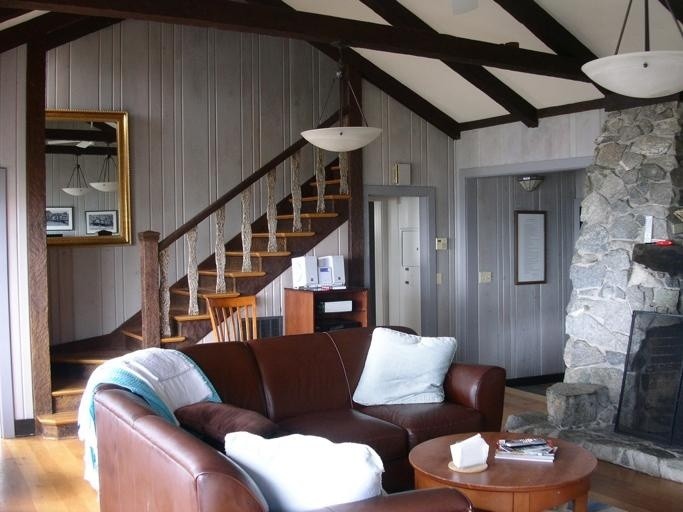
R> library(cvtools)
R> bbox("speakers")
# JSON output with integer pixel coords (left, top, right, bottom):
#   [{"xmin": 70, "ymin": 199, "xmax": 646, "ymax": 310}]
[
  {"xmin": 318, "ymin": 256, "xmax": 345, "ymax": 287},
  {"xmin": 291, "ymin": 256, "xmax": 319, "ymax": 289}
]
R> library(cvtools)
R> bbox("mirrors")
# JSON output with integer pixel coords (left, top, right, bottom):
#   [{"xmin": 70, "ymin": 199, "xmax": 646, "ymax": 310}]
[{"xmin": 42, "ymin": 105, "xmax": 133, "ymax": 250}]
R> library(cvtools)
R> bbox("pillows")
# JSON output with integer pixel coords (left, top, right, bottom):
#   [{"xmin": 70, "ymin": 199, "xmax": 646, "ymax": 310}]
[
  {"xmin": 172, "ymin": 403, "xmax": 278, "ymax": 449},
  {"xmin": 217, "ymin": 425, "xmax": 386, "ymax": 511},
  {"xmin": 348, "ymin": 324, "xmax": 458, "ymax": 412}
]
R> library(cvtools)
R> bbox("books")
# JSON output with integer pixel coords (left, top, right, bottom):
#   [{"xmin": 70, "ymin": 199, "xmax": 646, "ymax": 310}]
[{"xmin": 494, "ymin": 437, "xmax": 557, "ymax": 463}]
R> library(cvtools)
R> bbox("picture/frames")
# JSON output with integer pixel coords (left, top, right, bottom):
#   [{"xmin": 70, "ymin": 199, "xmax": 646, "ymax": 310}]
[
  {"xmin": 511, "ymin": 209, "xmax": 547, "ymax": 287},
  {"xmin": 84, "ymin": 208, "xmax": 119, "ymax": 236},
  {"xmin": 42, "ymin": 207, "xmax": 75, "ymax": 233}
]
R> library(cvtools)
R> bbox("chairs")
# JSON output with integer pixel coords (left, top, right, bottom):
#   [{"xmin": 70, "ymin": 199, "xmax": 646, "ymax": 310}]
[{"xmin": 203, "ymin": 294, "xmax": 257, "ymax": 343}]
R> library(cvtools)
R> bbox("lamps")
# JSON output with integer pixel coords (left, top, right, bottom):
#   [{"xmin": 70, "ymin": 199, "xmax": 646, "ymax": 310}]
[
  {"xmin": 517, "ymin": 174, "xmax": 544, "ymax": 192},
  {"xmin": 300, "ymin": 56, "xmax": 384, "ymax": 155},
  {"xmin": 86, "ymin": 142, "xmax": 118, "ymax": 196},
  {"xmin": 578, "ymin": 0, "xmax": 682, "ymax": 104},
  {"xmin": 57, "ymin": 149, "xmax": 88, "ymax": 198}
]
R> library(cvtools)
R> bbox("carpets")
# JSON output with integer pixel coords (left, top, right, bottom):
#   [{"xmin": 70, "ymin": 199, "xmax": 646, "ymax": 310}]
[{"xmin": 557, "ymin": 483, "xmax": 646, "ymax": 512}]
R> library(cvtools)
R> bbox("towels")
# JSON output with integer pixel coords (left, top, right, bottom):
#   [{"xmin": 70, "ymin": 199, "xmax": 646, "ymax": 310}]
[{"xmin": 69, "ymin": 342, "xmax": 221, "ymax": 493}]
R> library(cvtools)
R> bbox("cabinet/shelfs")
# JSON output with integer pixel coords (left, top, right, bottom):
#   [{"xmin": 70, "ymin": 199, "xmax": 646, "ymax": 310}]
[{"xmin": 282, "ymin": 284, "xmax": 371, "ymax": 336}]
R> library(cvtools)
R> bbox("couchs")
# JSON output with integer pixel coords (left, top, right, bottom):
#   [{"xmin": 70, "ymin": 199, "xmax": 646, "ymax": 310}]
[{"xmin": 92, "ymin": 325, "xmax": 509, "ymax": 512}]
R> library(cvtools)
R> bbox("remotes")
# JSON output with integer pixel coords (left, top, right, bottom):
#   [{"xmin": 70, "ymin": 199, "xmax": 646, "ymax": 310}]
[{"xmin": 505, "ymin": 437, "xmax": 548, "ymax": 448}]
